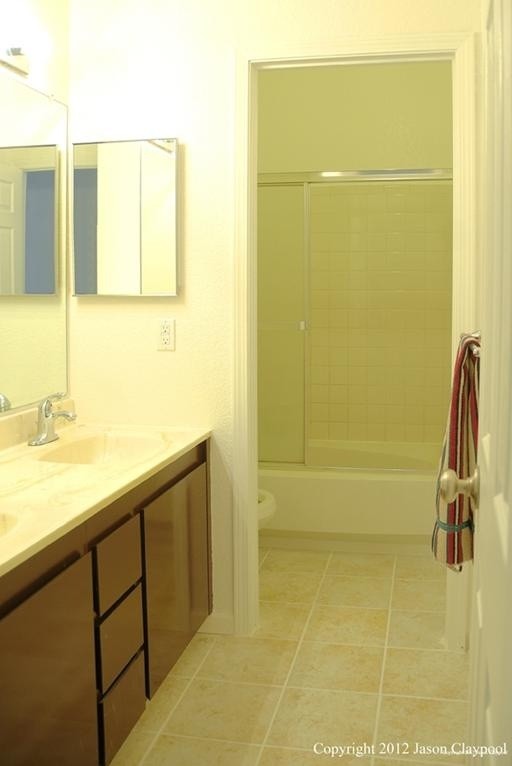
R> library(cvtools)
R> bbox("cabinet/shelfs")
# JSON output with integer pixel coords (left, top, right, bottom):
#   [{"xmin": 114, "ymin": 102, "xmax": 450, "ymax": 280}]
[{"xmin": 0, "ymin": 461, "xmax": 214, "ymax": 766}]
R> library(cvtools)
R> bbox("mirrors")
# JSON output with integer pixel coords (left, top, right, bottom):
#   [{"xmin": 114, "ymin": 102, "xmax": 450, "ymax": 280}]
[
  {"xmin": 0, "ymin": 145, "xmax": 61, "ymax": 296},
  {"xmin": 0, "ymin": 73, "xmax": 69, "ymax": 417},
  {"xmin": 74, "ymin": 137, "xmax": 178, "ymax": 298}
]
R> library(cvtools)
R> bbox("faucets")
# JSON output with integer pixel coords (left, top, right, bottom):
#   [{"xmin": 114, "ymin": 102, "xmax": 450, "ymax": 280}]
[
  {"xmin": 28, "ymin": 394, "xmax": 76, "ymax": 445},
  {"xmin": 0, "ymin": 394, "xmax": 9, "ymax": 412}
]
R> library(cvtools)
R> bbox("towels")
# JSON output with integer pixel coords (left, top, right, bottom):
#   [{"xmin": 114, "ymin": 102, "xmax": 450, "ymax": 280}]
[{"xmin": 431, "ymin": 334, "xmax": 479, "ymax": 573}]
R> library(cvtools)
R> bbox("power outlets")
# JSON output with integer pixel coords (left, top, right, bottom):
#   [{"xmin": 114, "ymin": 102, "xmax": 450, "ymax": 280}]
[{"xmin": 156, "ymin": 319, "xmax": 175, "ymax": 351}]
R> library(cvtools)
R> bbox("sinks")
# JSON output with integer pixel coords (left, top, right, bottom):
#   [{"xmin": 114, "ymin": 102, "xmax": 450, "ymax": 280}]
[{"xmin": 37, "ymin": 432, "xmax": 164, "ymax": 465}]
